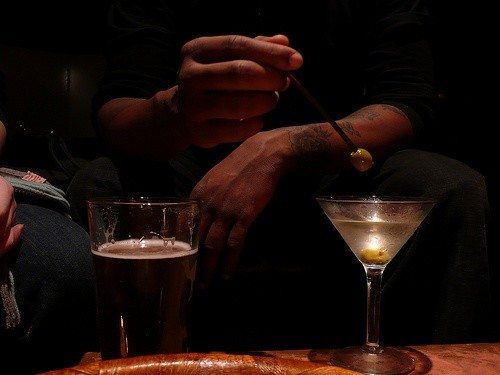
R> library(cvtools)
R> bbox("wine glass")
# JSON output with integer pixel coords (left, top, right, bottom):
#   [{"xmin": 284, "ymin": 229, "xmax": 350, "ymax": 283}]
[{"xmin": 316, "ymin": 195, "xmax": 438, "ymax": 375}]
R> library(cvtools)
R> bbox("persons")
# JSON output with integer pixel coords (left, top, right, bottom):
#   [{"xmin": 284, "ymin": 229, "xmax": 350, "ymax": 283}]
[
  {"xmin": 65, "ymin": 1, "xmax": 493, "ymax": 355},
  {"xmin": 1, "ymin": 118, "xmax": 99, "ymax": 375}
]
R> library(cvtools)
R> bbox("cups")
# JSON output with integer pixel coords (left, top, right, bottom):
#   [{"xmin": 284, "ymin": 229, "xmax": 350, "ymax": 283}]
[{"xmin": 86, "ymin": 195, "xmax": 205, "ymax": 359}]
